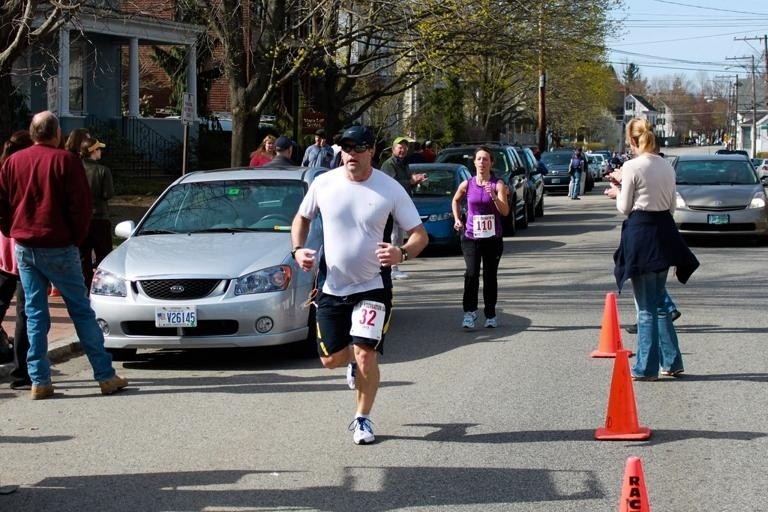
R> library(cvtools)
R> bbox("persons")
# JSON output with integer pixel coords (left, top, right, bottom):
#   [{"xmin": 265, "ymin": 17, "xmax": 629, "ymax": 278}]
[
  {"xmin": 605, "ymin": 167, "xmax": 681, "ymax": 334},
  {"xmin": 452, "ymin": 147, "xmax": 510, "ymax": 328},
  {"xmin": 604, "ymin": 117, "xmax": 700, "ymax": 381},
  {"xmin": 568, "ymin": 147, "xmax": 587, "ymax": 200},
  {"xmin": 0, "ymin": 111, "xmax": 128, "ymax": 400},
  {"xmin": 380, "ymin": 137, "xmax": 428, "ymax": 280},
  {"xmin": 250, "ymin": 129, "xmax": 435, "ymax": 171},
  {"xmin": 290, "ymin": 127, "xmax": 429, "ymax": 445}
]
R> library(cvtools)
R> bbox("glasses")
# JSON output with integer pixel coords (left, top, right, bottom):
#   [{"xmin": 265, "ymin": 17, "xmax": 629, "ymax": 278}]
[{"xmin": 340, "ymin": 143, "xmax": 369, "ymax": 153}]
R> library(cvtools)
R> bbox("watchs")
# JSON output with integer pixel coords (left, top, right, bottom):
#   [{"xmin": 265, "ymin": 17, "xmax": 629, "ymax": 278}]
[{"xmin": 291, "ymin": 247, "xmax": 301, "ymax": 259}]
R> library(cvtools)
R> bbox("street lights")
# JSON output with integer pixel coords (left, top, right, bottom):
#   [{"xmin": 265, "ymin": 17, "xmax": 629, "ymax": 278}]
[
  {"xmin": 703, "ymin": 95, "xmax": 730, "ymax": 143},
  {"xmin": 724, "ymin": 63, "xmax": 756, "ymax": 158},
  {"xmin": 714, "ymin": 75, "xmax": 739, "ymax": 151}
]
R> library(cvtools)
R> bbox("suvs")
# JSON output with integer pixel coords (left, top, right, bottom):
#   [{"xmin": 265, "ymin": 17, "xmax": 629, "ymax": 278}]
[
  {"xmin": 505, "ymin": 143, "xmax": 549, "ymax": 223},
  {"xmin": 540, "ymin": 146, "xmax": 595, "ymax": 193},
  {"xmin": 434, "ymin": 140, "xmax": 530, "ymax": 235}
]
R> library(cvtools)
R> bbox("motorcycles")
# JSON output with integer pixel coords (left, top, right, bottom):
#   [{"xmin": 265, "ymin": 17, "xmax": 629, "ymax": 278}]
[{"xmin": 688, "ymin": 138, "xmax": 694, "ymax": 145}]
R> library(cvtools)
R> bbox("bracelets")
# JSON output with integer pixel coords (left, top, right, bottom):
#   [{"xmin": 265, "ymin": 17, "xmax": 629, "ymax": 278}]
[
  {"xmin": 492, "ymin": 196, "xmax": 498, "ymax": 201},
  {"xmin": 400, "ymin": 248, "xmax": 408, "ymax": 262}
]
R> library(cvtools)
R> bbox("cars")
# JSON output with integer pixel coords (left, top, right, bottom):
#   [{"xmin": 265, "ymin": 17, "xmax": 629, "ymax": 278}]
[
  {"xmin": 670, "ymin": 149, "xmax": 768, "ymax": 243},
  {"xmin": 587, "ymin": 149, "xmax": 612, "ymax": 182},
  {"xmin": 88, "ymin": 165, "xmax": 330, "ymax": 360},
  {"xmin": 407, "ymin": 162, "xmax": 474, "ymax": 252}
]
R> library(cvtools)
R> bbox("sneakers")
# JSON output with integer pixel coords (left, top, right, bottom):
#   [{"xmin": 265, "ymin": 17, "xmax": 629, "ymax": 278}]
[
  {"xmin": 10, "ymin": 380, "xmax": 55, "ymax": 399},
  {"xmin": 672, "ymin": 310, "xmax": 680, "ymax": 320},
  {"xmin": 100, "ymin": 374, "xmax": 129, "ymax": 393},
  {"xmin": 391, "ymin": 266, "xmax": 409, "ymax": 280},
  {"xmin": 631, "ymin": 373, "xmax": 658, "ymax": 380},
  {"xmin": 484, "ymin": 318, "xmax": 497, "ymax": 328},
  {"xmin": 626, "ymin": 325, "xmax": 637, "ymax": 333},
  {"xmin": 462, "ymin": 309, "xmax": 479, "ymax": 328},
  {"xmin": 661, "ymin": 369, "xmax": 684, "ymax": 375},
  {"xmin": 347, "ymin": 361, "xmax": 357, "ymax": 389},
  {"xmin": 48, "ymin": 286, "xmax": 61, "ymax": 296},
  {"xmin": 348, "ymin": 417, "xmax": 375, "ymax": 445}
]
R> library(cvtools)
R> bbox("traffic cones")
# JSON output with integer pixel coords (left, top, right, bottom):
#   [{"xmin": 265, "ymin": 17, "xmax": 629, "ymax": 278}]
[
  {"xmin": 618, "ymin": 457, "xmax": 649, "ymax": 512},
  {"xmin": 588, "ymin": 292, "xmax": 634, "ymax": 360},
  {"xmin": 594, "ymin": 349, "xmax": 652, "ymax": 443}
]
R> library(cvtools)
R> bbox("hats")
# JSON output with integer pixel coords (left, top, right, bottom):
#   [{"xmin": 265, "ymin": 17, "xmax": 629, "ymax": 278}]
[
  {"xmin": 315, "ymin": 129, "xmax": 327, "ymax": 136},
  {"xmin": 275, "ymin": 136, "xmax": 296, "ymax": 148},
  {"xmin": 88, "ymin": 139, "xmax": 106, "ymax": 153},
  {"xmin": 393, "ymin": 137, "xmax": 409, "ymax": 147},
  {"xmin": 338, "ymin": 126, "xmax": 374, "ymax": 147}
]
